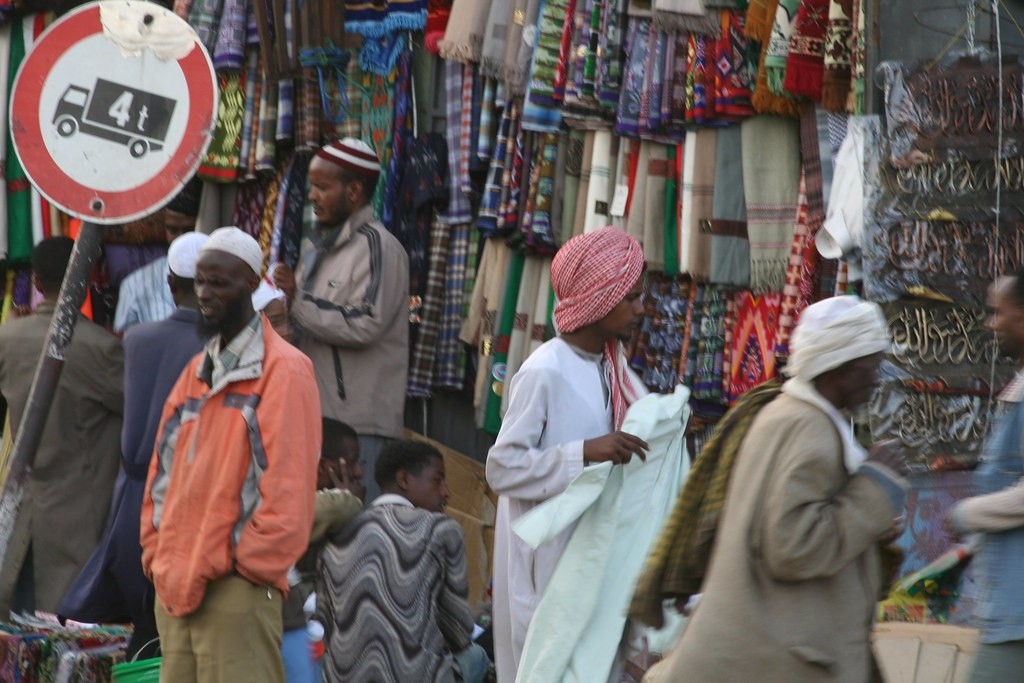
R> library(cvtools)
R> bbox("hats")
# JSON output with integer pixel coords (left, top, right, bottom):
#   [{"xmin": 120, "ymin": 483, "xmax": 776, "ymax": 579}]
[
  {"xmin": 201, "ymin": 224, "xmax": 264, "ymax": 277},
  {"xmin": 787, "ymin": 294, "xmax": 889, "ymax": 379},
  {"xmin": 167, "ymin": 231, "xmax": 210, "ymax": 279},
  {"xmin": 315, "ymin": 136, "xmax": 381, "ymax": 178},
  {"xmin": 548, "ymin": 227, "xmax": 646, "ymax": 336}
]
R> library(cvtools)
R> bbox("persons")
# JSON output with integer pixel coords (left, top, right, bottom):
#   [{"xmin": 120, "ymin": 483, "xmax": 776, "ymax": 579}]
[
  {"xmin": 946, "ymin": 272, "xmax": 1024, "ymax": 683},
  {"xmin": 486, "ymin": 228, "xmax": 694, "ymax": 683},
  {"xmin": 640, "ymin": 296, "xmax": 910, "ymax": 683},
  {"xmin": 0, "ymin": 135, "xmax": 487, "ymax": 683}
]
